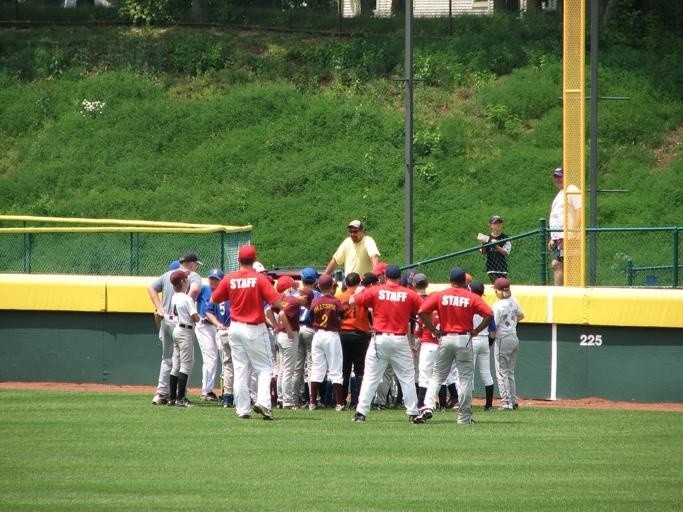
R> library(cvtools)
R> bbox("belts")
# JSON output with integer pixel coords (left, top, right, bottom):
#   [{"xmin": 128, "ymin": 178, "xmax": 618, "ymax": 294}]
[
  {"xmin": 376, "ymin": 332, "xmax": 406, "ymax": 335},
  {"xmin": 442, "ymin": 333, "xmax": 467, "ymax": 335},
  {"xmin": 179, "ymin": 325, "xmax": 192, "ymax": 329}
]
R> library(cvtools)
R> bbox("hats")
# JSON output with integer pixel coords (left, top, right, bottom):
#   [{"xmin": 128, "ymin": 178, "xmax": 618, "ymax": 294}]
[
  {"xmin": 277, "ymin": 268, "xmax": 337, "ymax": 310},
  {"xmin": 169, "ymin": 252, "xmax": 202, "ymax": 285},
  {"xmin": 491, "ymin": 278, "xmax": 510, "ymax": 291},
  {"xmin": 554, "ymin": 168, "xmax": 563, "ymax": 176},
  {"xmin": 348, "ymin": 220, "xmax": 363, "ymax": 230},
  {"xmin": 239, "ymin": 245, "xmax": 256, "ymax": 259},
  {"xmin": 346, "ymin": 263, "xmax": 428, "ymax": 287},
  {"xmin": 253, "ymin": 262, "xmax": 268, "ymax": 272},
  {"xmin": 490, "ymin": 216, "xmax": 502, "ymax": 223},
  {"xmin": 209, "ymin": 269, "xmax": 223, "ymax": 280},
  {"xmin": 450, "ymin": 267, "xmax": 483, "ymax": 294}
]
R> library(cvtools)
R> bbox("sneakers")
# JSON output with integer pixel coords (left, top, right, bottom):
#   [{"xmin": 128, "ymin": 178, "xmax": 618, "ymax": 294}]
[
  {"xmin": 409, "ymin": 408, "xmax": 433, "ymax": 423},
  {"xmin": 498, "ymin": 404, "xmax": 518, "ymax": 410},
  {"xmin": 200, "ymin": 393, "xmax": 233, "ymax": 407},
  {"xmin": 152, "ymin": 393, "xmax": 191, "ymax": 406},
  {"xmin": 458, "ymin": 419, "xmax": 475, "ymax": 424},
  {"xmin": 277, "ymin": 398, "xmax": 347, "ymax": 411},
  {"xmin": 352, "ymin": 415, "xmax": 365, "ymax": 421},
  {"xmin": 253, "ymin": 404, "xmax": 271, "ymax": 417}
]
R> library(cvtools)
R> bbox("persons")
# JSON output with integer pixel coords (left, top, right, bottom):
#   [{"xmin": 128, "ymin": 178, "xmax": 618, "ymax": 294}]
[
  {"xmin": 479, "ymin": 216, "xmax": 512, "ymax": 281},
  {"xmin": 149, "ymin": 245, "xmax": 523, "ymax": 423},
  {"xmin": 548, "ymin": 168, "xmax": 584, "ymax": 286},
  {"xmin": 325, "ymin": 220, "xmax": 380, "ymax": 274}
]
